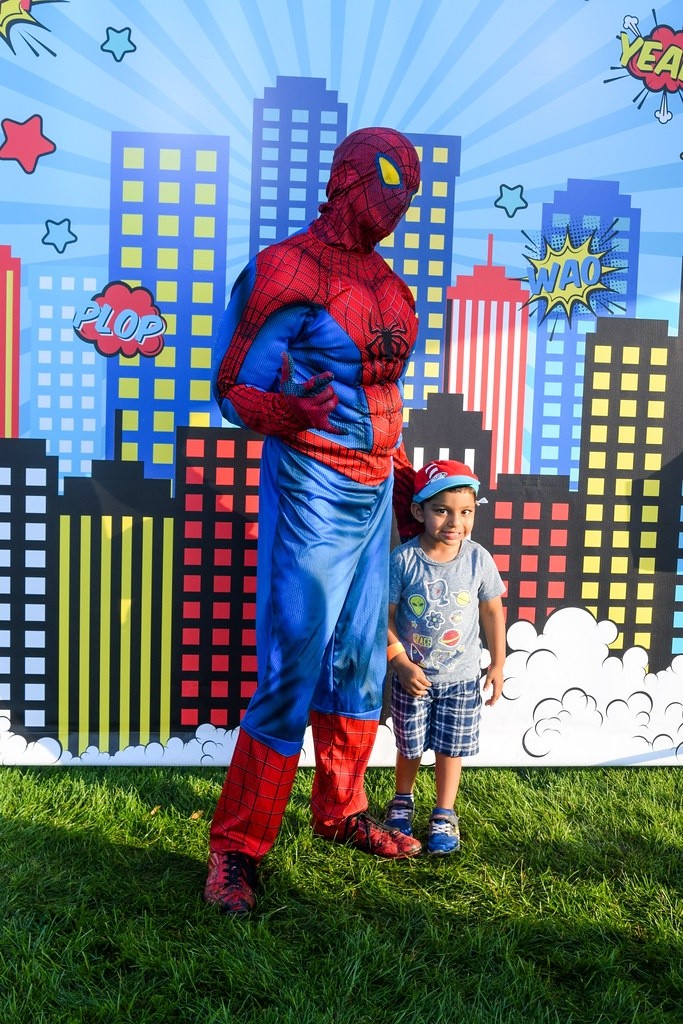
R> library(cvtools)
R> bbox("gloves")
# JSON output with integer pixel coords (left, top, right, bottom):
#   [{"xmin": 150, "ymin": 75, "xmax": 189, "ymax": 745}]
[{"xmin": 279, "ymin": 352, "xmax": 351, "ymax": 436}]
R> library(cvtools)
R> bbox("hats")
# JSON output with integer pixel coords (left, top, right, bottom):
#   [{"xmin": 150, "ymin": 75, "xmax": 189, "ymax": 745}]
[{"xmin": 412, "ymin": 460, "xmax": 479, "ymax": 504}]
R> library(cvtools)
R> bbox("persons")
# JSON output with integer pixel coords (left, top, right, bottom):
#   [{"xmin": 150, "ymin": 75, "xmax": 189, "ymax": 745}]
[
  {"xmin": 206, "ymin": 124, "xmax": 425, "ymax": 911},
  {"xmin": 385, "ymin": 460, "xmax": 508, "ymax": 855}
]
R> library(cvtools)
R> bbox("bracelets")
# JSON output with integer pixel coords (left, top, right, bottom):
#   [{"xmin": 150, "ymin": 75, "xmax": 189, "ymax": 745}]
[{"xmin": 387, "ymin": 642, "xmax": 405, "ymax": 661}]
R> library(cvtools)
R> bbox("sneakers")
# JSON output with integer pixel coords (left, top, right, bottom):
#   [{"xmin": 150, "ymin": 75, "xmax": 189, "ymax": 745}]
[
  {"xmin": 427, "ymin": 807, "xmax": 459, "ymax": 854},
  {"xmin": 311, "ymin": 811, "xmax": 423, "ymax": 859},
  {"xmin": 385, "ymin": 795, "xmax": 416, "ymax": 838},
  {"xmin": 201, "ymin": 850, "xmax": 261, "ymax": 914}
]
